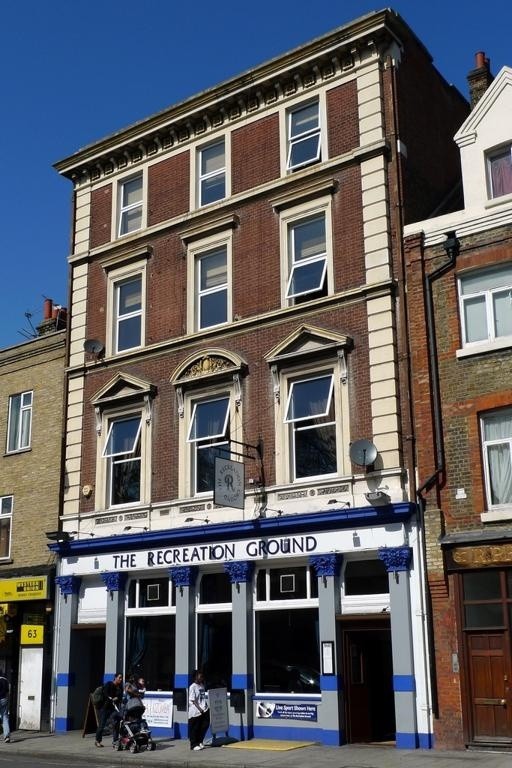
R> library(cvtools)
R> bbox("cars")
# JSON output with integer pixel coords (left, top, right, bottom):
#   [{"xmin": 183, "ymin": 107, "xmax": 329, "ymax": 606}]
[{"xmin": 260, "ymin": 660, "xmax": 322, "ymax": 693}]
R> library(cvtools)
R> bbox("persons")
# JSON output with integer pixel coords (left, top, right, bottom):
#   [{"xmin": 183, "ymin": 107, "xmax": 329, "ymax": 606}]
[
  {"xmin": 0, "ymin": 666, "xmax": 12, "ymax": 745},
  {"xmin": 121, "ymin": 669, "xmax": 145, "ymax": 708},
  {"xmin": 135, "ymin": 677, "xmax": 147, "ymax": 700},
  {"xmin": 188, "ymin": 669, "xmax": 211, "ymax": 753},
  {"xmin": 95, "ymin": 671, "xmax": 123, "ymax": 748}
]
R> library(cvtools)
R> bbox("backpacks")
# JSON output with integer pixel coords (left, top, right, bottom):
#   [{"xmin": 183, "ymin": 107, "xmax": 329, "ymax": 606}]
[{"xmin": 90, "ymin": 686, "xmax": 103, "ymax": 706}]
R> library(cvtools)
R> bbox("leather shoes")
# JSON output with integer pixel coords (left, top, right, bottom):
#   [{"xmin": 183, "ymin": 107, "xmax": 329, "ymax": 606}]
[{"xmin": 94, "ymin": 741, "xmax": 104, "ymax": 747}]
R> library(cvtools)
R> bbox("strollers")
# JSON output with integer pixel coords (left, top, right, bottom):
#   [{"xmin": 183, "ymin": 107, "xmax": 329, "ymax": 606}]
[{"xmin": 109, "ymin": 690, "xmax": 156, "ymax": 755}]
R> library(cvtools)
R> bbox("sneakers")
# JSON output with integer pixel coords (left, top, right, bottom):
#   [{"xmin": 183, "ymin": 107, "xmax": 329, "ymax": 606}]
[
  {"xmin": 192, "ymin": 745, "xmax": 202, "ymax": 751},
  {"xmin": 5, "ymin": 736, "xmax": 11, "ymax": 742},
  {"xmin": 199, "ymin": 743, "xmax": 205, "ymax": 749}
]
